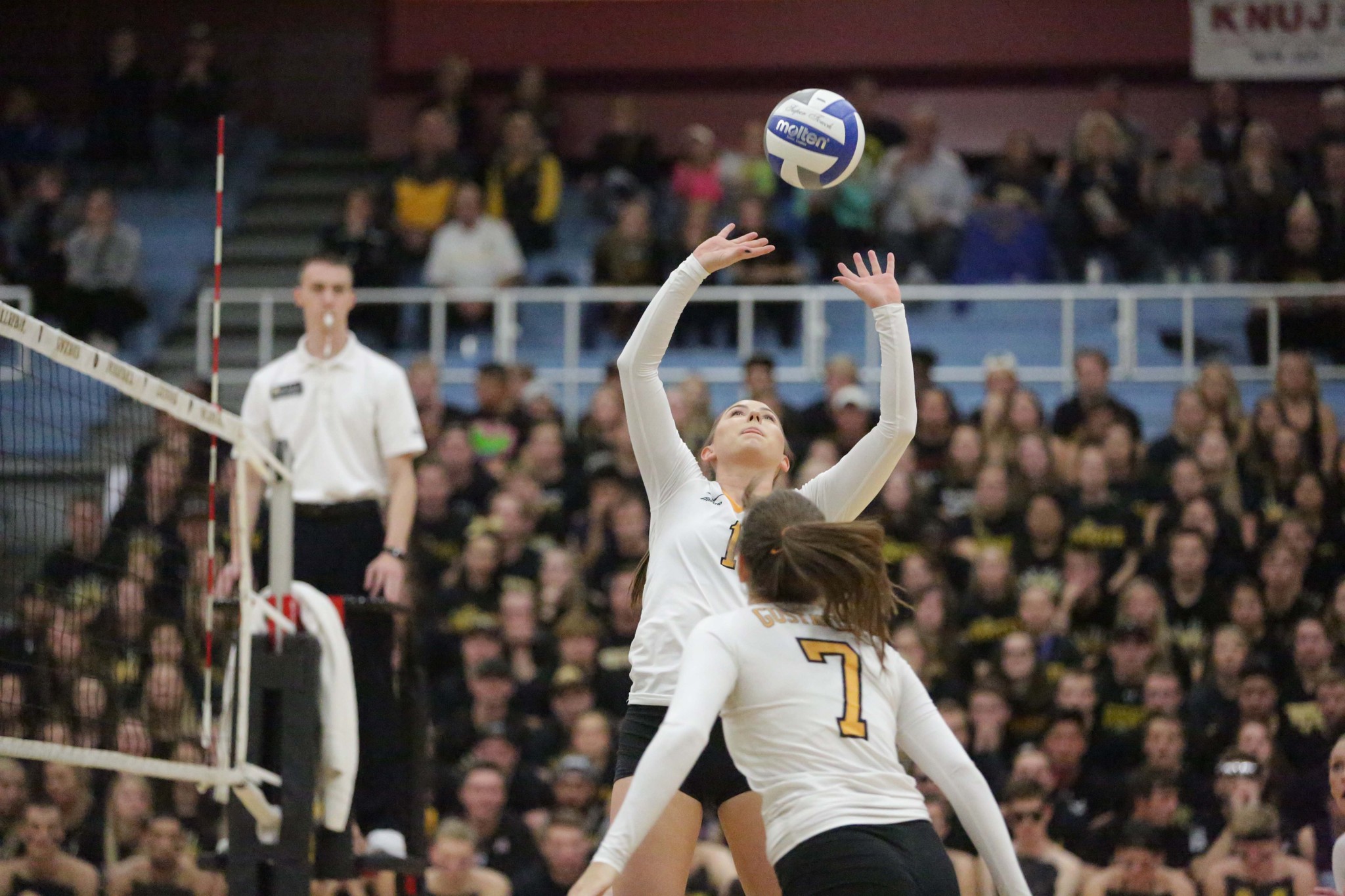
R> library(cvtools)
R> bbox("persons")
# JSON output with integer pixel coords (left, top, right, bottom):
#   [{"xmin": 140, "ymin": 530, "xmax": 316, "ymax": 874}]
[
  {"xmin": 566, "ymin": 485, "xmax": 1036, "ymax": 896},
  {"xmin": 607, "ymin": 222, "xmax": 920, "ymax": 896},
  {"xmin": 215, "ymin": 253, "xmax": 427, "ymax": 863},
  {"xmin": 0, "ymin": 1, "xmax": 1345, "ymax": 896}
]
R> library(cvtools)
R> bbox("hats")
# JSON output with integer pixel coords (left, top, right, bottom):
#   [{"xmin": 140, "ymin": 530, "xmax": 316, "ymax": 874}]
[
  {"xmin": 557, "ymin": 754, "xmax": 591, "ymax": 772},
  {"xmin": 831, "ymin": 385, "xmax": 873, "ymax": 411},
  {"xmin": 365, "ymin": 829, "xmax": 407, "ymax": 860},
  {"xmin": 983, "ymin": 352, "xmax": 1016, "ymax": 375},
  {"xmin": 685, "ymin": 123, "xmax": 713, "ymax": 144},
  {"xmin": 547, "ymin": 660, "xmax": 591, "ymax": 693}
]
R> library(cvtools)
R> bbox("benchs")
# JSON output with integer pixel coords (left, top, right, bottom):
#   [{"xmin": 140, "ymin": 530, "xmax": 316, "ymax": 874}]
[
  {"xmin": 389, "ymin": 184, "xmax": 1345, "ymax": 439},
  {"xmin": 0, "ymin": 117, "xmax": 280, "ymax": 459}
]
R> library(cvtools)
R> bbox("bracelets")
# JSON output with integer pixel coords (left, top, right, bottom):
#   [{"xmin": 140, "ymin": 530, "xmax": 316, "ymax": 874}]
[{"xmin": 381, "ymin": 543, "xmax": 410, "ymax": 562}]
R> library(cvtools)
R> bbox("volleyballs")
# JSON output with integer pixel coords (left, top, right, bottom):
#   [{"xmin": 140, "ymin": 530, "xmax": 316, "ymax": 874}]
[{"xmin": 762, "ymin": 86, "xmax": 867, "ymax": 190}]
